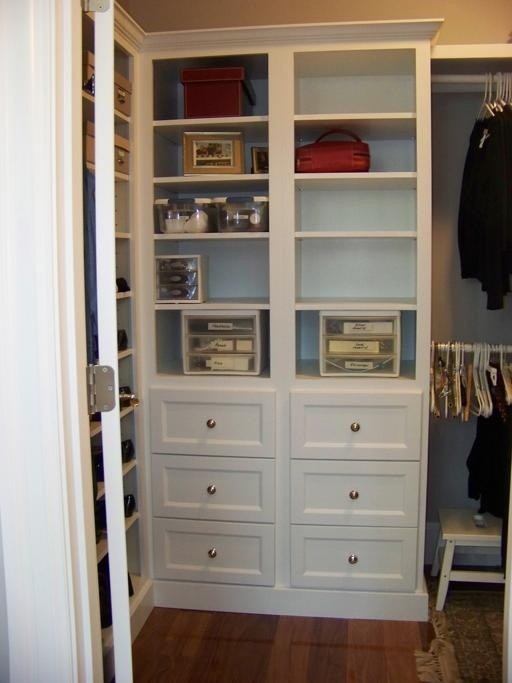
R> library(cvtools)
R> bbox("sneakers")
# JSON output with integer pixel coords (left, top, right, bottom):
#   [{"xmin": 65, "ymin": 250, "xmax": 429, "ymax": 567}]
[{"xmin": 88, "ymin": 275, "xmax": 136, "ymax": 627}]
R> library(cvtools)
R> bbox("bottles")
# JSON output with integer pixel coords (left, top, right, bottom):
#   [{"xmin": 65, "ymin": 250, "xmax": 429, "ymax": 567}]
[
  {"xmin": 166, "ymin": 198, "xmax": 193, "ymax": 221},
  {"xmin": 222, "ymin": 197, "xmax": 253, "ymax": 227}
]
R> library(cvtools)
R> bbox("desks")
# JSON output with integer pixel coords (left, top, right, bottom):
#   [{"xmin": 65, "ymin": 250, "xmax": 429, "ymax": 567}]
[{"xmin": 143, "ymin": 386, "xmax": 288, "ymax": 616}]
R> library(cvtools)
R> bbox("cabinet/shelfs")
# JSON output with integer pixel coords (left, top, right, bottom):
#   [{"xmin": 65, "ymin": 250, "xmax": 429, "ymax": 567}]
[
  {"xmin": 143, "ymin": 40, "xmax": 286, "ymax": 386},
  {"xmin": 2, "ymin": 0, "xmax": 146, "ymax": 683},
  {"xmin": 288, "ymin": 385, "xmax": 428, "ymax": 622},
  {"xmin": 285, "ymin": 39, "xmax": 432, "ymax": 385}
]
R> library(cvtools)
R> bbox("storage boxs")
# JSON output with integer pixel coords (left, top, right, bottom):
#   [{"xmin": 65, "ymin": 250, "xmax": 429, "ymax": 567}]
[
  {"xmin": 180, "ymin": 66, "xmax": 256, "ymax": 118},
  {"xmin": 181, "ymin": 310, "xmax": 261, "ymax": 376},
  {"xmin": 318, "ymin": 310, "xmax": 401, "ymax": 378},
  {"xmin": 155, "ymin": 254, "xmax": 207, "ymax": 304},
  {"xmin": 82, "ymin": 50, "xmax": 95, "ymax": 90},
  {"xmin": 155, "ymin": 196, "xmax": 214, "ymax": 234},
  {"xmin": 113, "ymin": 71, "xmax": 133, "ymax": 116},
  {"xmin": 83, "ymin": 120, "xmax": 95, "ymax": 165},
  {"xmin": 211, "ymin": 197, "xmax": 269, "ymax": 232},
  {"xmin": 114, "ymin": 133, "xmax": 129, "ymax": 174}
]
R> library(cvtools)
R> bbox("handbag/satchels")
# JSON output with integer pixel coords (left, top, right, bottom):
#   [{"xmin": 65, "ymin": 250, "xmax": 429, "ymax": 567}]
[{"xmin": 295, "ymin": 127, "xmax": 370, "ymax": 172}]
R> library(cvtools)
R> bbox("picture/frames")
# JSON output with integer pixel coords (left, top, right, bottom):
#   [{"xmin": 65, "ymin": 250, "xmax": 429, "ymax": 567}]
[
  {"xmin": 182, "ymin": 131, "xmax": 245, "ymax": 176},
  {"xmin": 251, "ymin": 146, "xmax": 269, "ymax": 173}
]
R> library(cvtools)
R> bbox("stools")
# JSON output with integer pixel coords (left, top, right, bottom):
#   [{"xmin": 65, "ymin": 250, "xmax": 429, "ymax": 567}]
[{"xmin": 431, "ymin": 510, "xmax": 506, "ymax": 611}]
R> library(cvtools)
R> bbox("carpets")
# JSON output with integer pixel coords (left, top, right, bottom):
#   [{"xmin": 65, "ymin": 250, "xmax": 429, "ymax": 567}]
[{"xmin": 413, "ymin": 592, "xmax": 505, "ymax": 683}]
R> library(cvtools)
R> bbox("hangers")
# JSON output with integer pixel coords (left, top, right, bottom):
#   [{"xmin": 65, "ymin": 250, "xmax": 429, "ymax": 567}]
[
  {"xmin": 474, "ymin": 71, "xmax": 511, "ymax": 120},
  {"xmin": 429, "ymin": 340, "xmax": 512, "ymax": 422}
]
